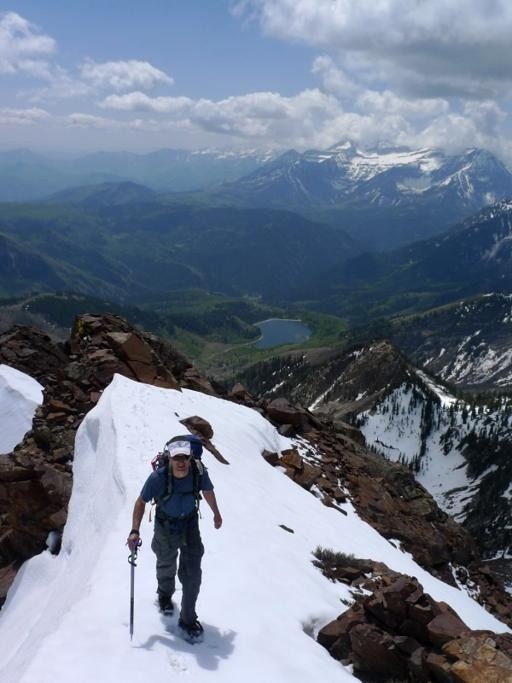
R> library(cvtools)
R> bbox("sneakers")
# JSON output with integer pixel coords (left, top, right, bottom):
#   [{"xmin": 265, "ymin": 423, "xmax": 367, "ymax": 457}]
[
  {"xmin": 158, "ymin": 593, "xmax": 174, "ymax": 616},
  {"xmin": 178, "ymin": 613, "xmax": 204, "ymax": 637}
]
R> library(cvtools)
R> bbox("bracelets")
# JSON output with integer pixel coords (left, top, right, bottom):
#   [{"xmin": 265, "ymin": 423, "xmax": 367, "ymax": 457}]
[{"xmin": 130, "ymin": 529, "xmax": 139, "ymax": 536}]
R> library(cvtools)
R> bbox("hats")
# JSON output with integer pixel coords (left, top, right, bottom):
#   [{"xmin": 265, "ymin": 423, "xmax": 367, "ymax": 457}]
[{"xmin": 167, "ymin": 440, "xmax": 191, "ymax": 458}]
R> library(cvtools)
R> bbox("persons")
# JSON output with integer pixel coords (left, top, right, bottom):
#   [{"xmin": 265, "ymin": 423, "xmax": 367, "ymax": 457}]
[{"xmin": 127, "ymin": 436, "xmax": 222, "ymax": 637}]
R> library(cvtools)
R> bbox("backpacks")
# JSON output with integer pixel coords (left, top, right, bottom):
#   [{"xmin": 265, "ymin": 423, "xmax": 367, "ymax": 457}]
[{"xmin": 150, "ymin": 435, "xmax": 204, "ymax": 523}]
